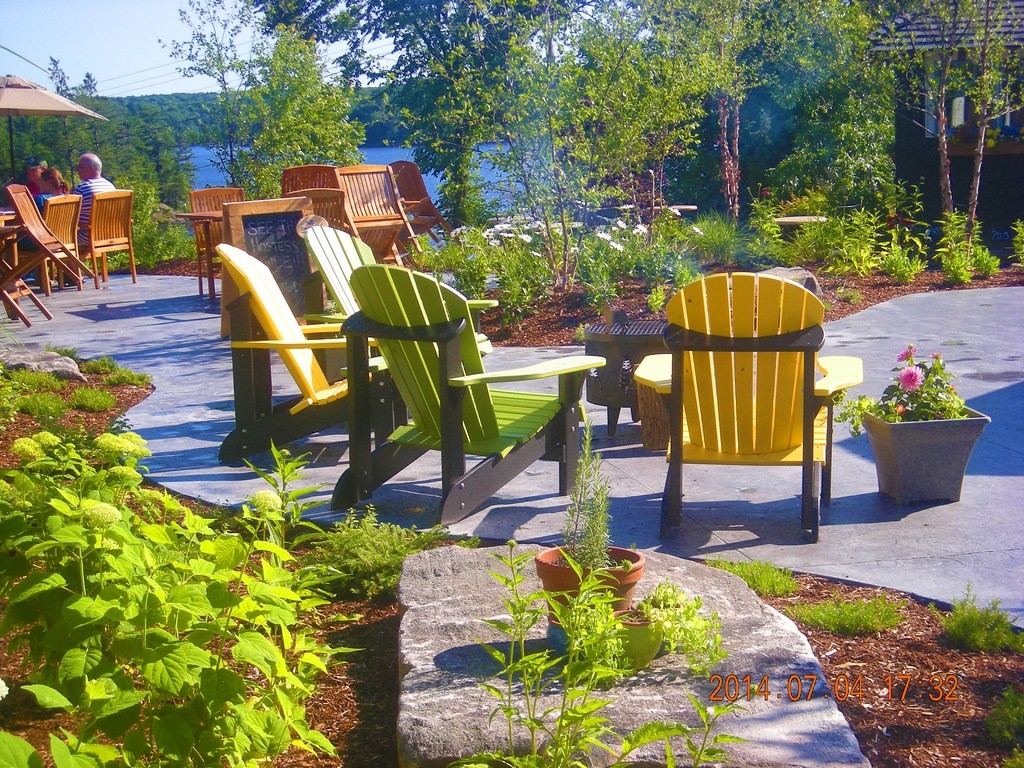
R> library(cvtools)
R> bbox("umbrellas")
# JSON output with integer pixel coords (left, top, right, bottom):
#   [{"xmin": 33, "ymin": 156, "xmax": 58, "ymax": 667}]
[{"xmin": 0, "ymin": 73, "xmax": 110, "ymax": 184}]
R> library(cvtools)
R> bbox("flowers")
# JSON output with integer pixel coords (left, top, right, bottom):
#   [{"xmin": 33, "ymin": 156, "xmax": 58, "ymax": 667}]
[{"xmin": 830, "ymin": 342, "xmax": 968, "ymax": 437}]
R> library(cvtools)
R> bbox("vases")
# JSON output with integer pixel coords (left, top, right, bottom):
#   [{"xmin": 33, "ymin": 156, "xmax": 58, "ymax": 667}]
[{"xmin": 859, "ymin": 404, "xmax": 992, "ymax": 504}]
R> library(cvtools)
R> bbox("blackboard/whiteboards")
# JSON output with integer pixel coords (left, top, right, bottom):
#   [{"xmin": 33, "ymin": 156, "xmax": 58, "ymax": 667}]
[{"xmin": 221, "ymin": 197, "xmax": 318, "ymax": 338}]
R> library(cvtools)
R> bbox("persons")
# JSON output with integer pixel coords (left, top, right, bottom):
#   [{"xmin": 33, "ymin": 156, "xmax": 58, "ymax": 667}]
[{"xmin": 16, "ymin": 153, "xmax": 117, "ymax": 286}]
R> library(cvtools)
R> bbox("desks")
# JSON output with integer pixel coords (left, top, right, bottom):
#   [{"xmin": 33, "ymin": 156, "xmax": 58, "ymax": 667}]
[
  {"xmin": 0, "ymin": 213, "xmax": 19, "ymax": 320},
  {"xmin": 174, "ymin": 200, "xmax": 421, "ymax": 223},
  {"xmin": 646, "ymin": 205, "xmax": 698, "ymax": 211},
  {"xmin": 584, "ymin": 319, "xmax": 667, "ymax": 439},
  {"xmin": 772, "ymin": 215, "xmax": 826, "ymax": 245},
  {"xmin": 529, "ymin": 221, "xmax": 583, "ymax": 231}
]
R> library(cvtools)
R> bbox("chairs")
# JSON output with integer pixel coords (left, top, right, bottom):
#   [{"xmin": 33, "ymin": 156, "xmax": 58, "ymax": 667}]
[
  {"xmin": 284, "ymin": 188, "xmax": 345, "ymax": 231},
  {"xmin": 0, "ymin": 184, "xmax": 95, "ymax": 329},
  {"xmin": 386, "ymin": 161, "xmax": 462, "ymax": 272},
  {"xmin": 303, "ymin": 225, "xmax": 499, "ymax": 375},
  {"xmin": 633, "ymin": 272, "xmax": 863, "ymax": 543},
  {"xmin": 333, "ymin": 165, "xmax": 425, "ymax": 253},
  {"xmin": 330, "ymin": 265, "xmax": 606, "ymax": 526},
  {"xmin": 281, "ymin": 164, "xmax": 338, "ymax": 198},
  {"xmin": 188, "ymin": 187, "xmax": 245, "ymax": 298},
  {"xmin": 78, "ymin": 189, "xmax": 137, "ymax": 289},
  {"xmin": 215, "ymin": 241, "xmax": 378, "ymax": 460},
  {"xmin": 18, "ymin": 194, "xmax": 83, "ymax": 297}
]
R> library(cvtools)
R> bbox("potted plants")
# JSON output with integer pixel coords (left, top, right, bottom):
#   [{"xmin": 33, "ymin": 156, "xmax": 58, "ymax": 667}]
[
  {"xmin": 533, "ymin": 399, "xmax": 644, "ymax": 621},
  {"xmin": 611, "ymin": 575, "xmax": 685, "ymax": 678}
]
